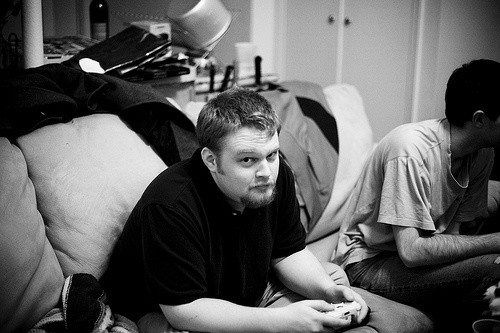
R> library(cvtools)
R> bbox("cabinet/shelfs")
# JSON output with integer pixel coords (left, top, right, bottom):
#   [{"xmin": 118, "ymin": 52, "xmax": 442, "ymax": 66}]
[{"xmin": 278, "ymin": 0, "xmax": 418, "ymax": 152}]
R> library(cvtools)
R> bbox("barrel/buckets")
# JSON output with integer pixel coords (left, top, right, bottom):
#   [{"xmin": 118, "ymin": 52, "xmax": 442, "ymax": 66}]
[{"xmin": 166, "ymin": 1, "xmax": 232, "ymax": 49}]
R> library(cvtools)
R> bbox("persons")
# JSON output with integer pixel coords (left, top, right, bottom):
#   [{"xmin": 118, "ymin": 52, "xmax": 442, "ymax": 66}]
[
  {"xmin": 99, "ymin": 85, "xmax": 371, "ymax": 333},
  {"xmin": 329, "ymin": 58, "xmax": 500, "ymax": 320}
]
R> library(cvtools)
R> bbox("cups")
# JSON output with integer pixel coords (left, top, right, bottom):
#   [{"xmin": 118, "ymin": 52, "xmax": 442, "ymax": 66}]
[{"xmin": 472, "ymin": 320, "xmax": 500, "ymax": 333}]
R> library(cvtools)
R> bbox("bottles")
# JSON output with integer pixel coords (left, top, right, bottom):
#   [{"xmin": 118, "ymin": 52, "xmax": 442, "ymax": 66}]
[{"xmin": 89, "ymin": 0, "xmax": 110, "ymax": 43}]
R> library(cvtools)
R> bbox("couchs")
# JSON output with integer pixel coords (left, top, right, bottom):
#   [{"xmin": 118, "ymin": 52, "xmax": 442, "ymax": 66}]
[{"xmin": 0, "ymin": 81, "xmax": 381, "ymax": 333}]
